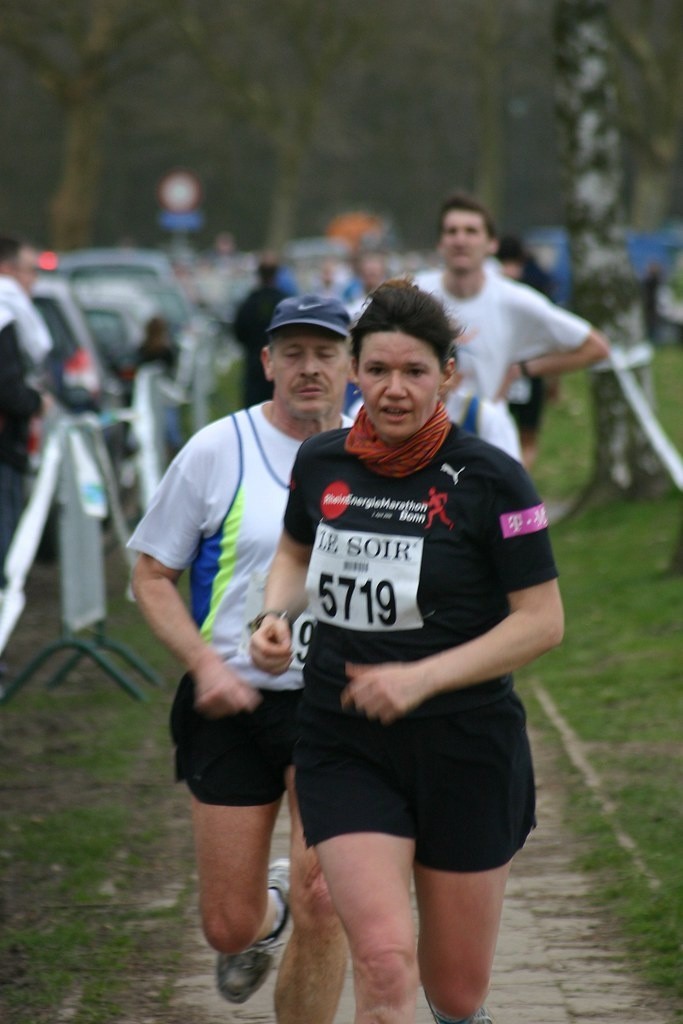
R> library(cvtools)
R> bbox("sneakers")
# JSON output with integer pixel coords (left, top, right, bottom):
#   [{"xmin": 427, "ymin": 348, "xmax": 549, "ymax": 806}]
[{"xmin": 215, "ymin": 860, "xmax": 295, "ymax": 1003}]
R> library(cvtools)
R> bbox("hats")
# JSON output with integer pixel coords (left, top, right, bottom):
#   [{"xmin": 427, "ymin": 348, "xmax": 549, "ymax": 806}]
[{"xmin": 265, "ymin": 294, "xmax": 350, "ymax": 336}]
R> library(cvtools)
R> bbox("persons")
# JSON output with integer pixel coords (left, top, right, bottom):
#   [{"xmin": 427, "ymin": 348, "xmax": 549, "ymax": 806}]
[
  {"xmin": 250, "ymin": 279, "xmax": 567, "ymax": 1023},
  {"xmin": 126, "ymin": 293, "xmax": 354, "ymax": 1024},
  {"xmin": 0, "ymin": 232, "xmax": 181, "ymax": 677},
  {"xmin": 231, "ymin": 196, "xmax": 610, "ymax": 463}
]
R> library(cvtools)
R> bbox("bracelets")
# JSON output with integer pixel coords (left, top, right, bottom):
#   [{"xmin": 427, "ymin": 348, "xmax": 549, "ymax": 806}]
[{"xmin": 249, "ymin": 608, "xmax": 289, "ymax": 635}]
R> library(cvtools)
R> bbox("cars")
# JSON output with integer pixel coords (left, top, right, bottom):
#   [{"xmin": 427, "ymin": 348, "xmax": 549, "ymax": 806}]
[{"xmin": 27, "ymin": 245, "xmax": 263, "ymax": 524}]
